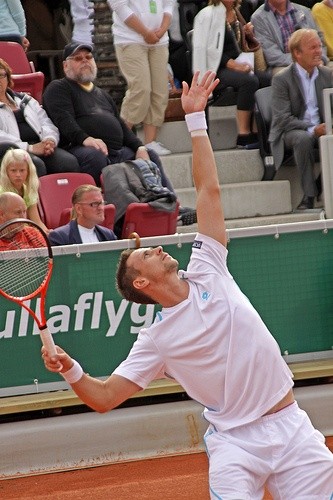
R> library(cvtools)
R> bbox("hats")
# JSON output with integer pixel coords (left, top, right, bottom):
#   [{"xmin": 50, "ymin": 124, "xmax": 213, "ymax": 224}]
[{"xmin": 62, "ymin": 40, "xmax": 93, "ymax": 61}]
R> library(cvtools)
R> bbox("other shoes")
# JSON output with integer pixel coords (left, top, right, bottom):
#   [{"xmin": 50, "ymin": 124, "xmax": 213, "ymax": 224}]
[
  {"xmin": 236, "ymin": 133, "xmax": 260, "ymax": 149},
  {"xmin": 314, "ymin": 173, "xmax": 322, "ymax": 196},
  {"xmin": 296, "ymin": 194, "xmax": 315, "ymax": 209},
  {"xmin": 177, "ymin": 207, "xmax": 197, "ymax": 226}
]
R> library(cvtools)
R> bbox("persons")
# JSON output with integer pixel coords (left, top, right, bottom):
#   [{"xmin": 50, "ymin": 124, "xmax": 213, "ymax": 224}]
[
  {"xmin": 42, "ymin": 69, "xmax": 333, "ymax": 500},
  {"xmin": 0, "ymin": 0, "xmax": 333, "ymax": 251}
]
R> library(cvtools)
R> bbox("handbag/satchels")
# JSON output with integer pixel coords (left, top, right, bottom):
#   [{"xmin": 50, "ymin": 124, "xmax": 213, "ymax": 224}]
[{"xmin": 234, "ymin": 6, "xmax": 267, "ymax": 72}]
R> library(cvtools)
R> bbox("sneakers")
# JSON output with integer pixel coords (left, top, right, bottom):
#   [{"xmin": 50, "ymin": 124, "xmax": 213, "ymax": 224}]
[{"xmin": 145, "ymin": 140, "xmax": 172, "ymax": 156}]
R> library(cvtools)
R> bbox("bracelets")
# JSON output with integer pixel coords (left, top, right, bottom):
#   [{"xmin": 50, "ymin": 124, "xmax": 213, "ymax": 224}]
[
  {"xmin": 185, "ymin": 111, "xmax": 208, "ymax": 137},
  {"xmin": 58, "ymin": 359, "xmax": 83, "ymax": 384}
]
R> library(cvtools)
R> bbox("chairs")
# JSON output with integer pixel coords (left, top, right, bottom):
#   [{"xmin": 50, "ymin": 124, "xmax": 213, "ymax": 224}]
[
  {"xmin": 0, "ymin": 39, "xmax": 180, "ymax": 240},
  {"xmin": 185, "ymin": 27, "xmax": 333, "ymax": 201}
]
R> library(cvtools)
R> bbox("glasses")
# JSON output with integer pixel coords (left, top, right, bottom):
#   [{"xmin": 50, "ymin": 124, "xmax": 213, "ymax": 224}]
[
  {"xmin": 68, "ymin": 54, "xmax": 93, "ymax": 61},
  {"xmin": 75, "ymin": 201, "xmax": 108, "ymax": 208},
  {"xmin": 0, "ymin": 73, "xmax": 7, "ymax": 79}
]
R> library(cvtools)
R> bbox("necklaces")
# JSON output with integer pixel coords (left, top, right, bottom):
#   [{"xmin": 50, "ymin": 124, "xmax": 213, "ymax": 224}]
[{"xmin": 226, "ymin": 8, "xmax": 242, "ymax": 42}]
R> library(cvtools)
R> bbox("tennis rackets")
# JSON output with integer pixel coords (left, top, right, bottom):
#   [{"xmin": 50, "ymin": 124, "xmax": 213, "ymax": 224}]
[{"xmin": 0, "ymin": 217, "xmax": 64, "ymax": 373}]
